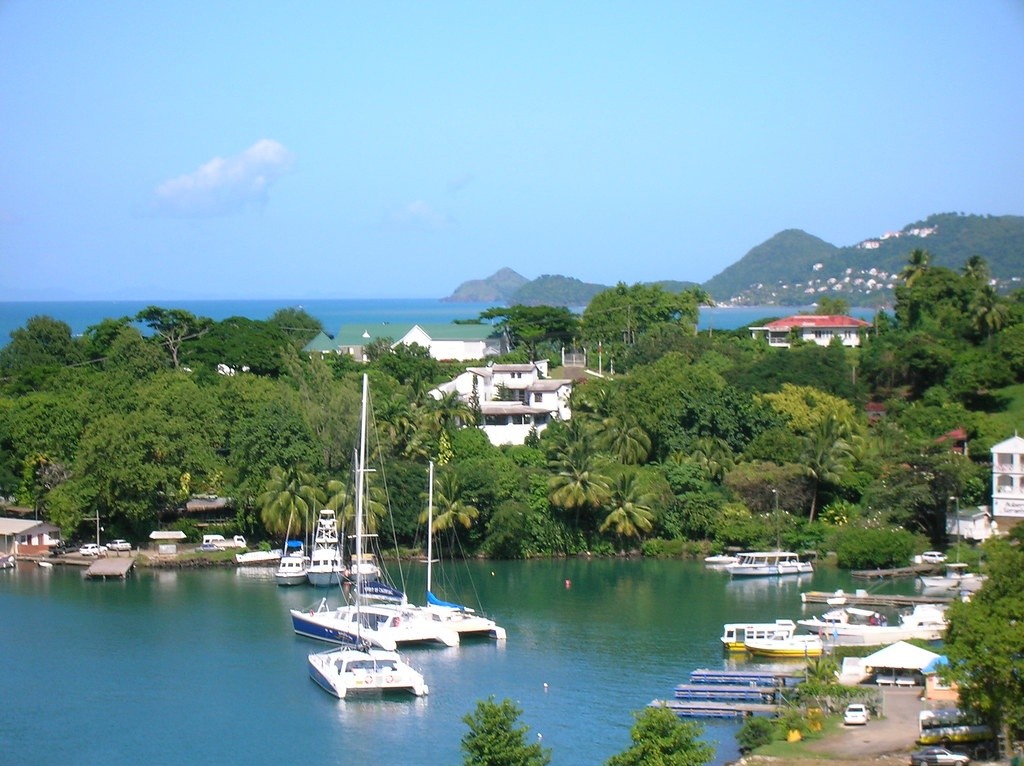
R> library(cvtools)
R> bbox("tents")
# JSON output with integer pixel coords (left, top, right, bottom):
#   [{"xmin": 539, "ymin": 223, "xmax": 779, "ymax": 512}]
[{"xmin": 858, "ymin": 641, "xmax": 941, "ymax": 684}]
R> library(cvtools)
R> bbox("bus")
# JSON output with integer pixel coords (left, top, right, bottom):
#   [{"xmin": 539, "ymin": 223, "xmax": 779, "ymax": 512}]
[{"xmin": 917, "ymin": 710, "xmax": 997, "ymax": 743}]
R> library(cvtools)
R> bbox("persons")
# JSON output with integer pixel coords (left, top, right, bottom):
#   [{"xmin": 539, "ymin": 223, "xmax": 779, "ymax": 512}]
[{"xmin": 870, "ymin": 610, "xmax": 888, "ymax": 627}]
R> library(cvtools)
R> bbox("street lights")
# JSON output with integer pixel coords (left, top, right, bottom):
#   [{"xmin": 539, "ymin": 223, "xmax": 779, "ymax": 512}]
[
  {"xmin": 950, "ymin": 497, "xmax": 961, "ymax": 564},
  {"xmin": 771, "ymin": 489, "xmax": 781, "ymax": 549}
]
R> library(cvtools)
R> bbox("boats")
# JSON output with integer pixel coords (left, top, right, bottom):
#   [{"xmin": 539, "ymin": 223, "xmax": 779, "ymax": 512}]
[
  {"xmin": 704, "ymin": 552, "xmax": 733, "ymax": 564},
  {"xmin": 725, "ymin": 552, "xmax": 814, "ymax": 578},
  {"xmin": 720, "ymin": 571, "xmax": 961, "ymax": 659}
]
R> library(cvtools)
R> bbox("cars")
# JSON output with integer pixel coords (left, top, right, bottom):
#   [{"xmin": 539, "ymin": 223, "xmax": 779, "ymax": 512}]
[
  {"xmin": 844, "ymin": 702, "xmax": 871, "ymax": 726},
  {"xmin": 909, "ymin": 746, "xmax": 971, "ymax": 766},
  {"xmin": 80, "ymin": 543, "xmax": 112, "ymax": 556},
  {"xmin": 106, "ymin": 539, "xmax": 133, "ymax": 550},
  {"xmin": 195, "ymin": 544, "xmax": 225, "ymax": 553},
  {"xmin": 48, "ymin": 541, "xmax": 78, "ymax": 556}
]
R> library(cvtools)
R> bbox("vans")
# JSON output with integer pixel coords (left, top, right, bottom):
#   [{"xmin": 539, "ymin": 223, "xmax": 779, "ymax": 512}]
[
  {"xmin": 202, "ymin": 534, "xmax": 226, "ymax": 546},
  {"xmin": 922, "ymin": 550, "xmax": 948, "ymax": 563}
]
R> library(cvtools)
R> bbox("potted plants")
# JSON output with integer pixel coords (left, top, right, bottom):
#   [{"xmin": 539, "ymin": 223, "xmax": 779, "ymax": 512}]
[
  {"xmin": 800, "ymin": 677, "xmax": 823, "ymax": 733},
  {"xmin": 778, "ymin": 709, "xmax": 805, "ymax": 742}
]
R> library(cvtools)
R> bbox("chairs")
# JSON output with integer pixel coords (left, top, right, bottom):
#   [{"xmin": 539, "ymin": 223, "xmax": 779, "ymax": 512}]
[
  {"xmin": 352, "ymin": 667, "xmax": 369, "ymax": 682},
  {"xmin": 371, "ymin": 667, "xmax": 391, "ymax": 679}
]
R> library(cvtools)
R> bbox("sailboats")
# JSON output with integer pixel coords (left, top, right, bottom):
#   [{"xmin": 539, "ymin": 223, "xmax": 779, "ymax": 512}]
[{"xmin": 240, "ymin": 372, "xmax": 507, "ymax": 700}]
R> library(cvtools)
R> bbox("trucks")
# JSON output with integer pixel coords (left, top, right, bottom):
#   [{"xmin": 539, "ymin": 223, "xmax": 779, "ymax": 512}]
[{"xmin": 214, "ymin": 535, "xmax": 248, "ymax": 549}]
